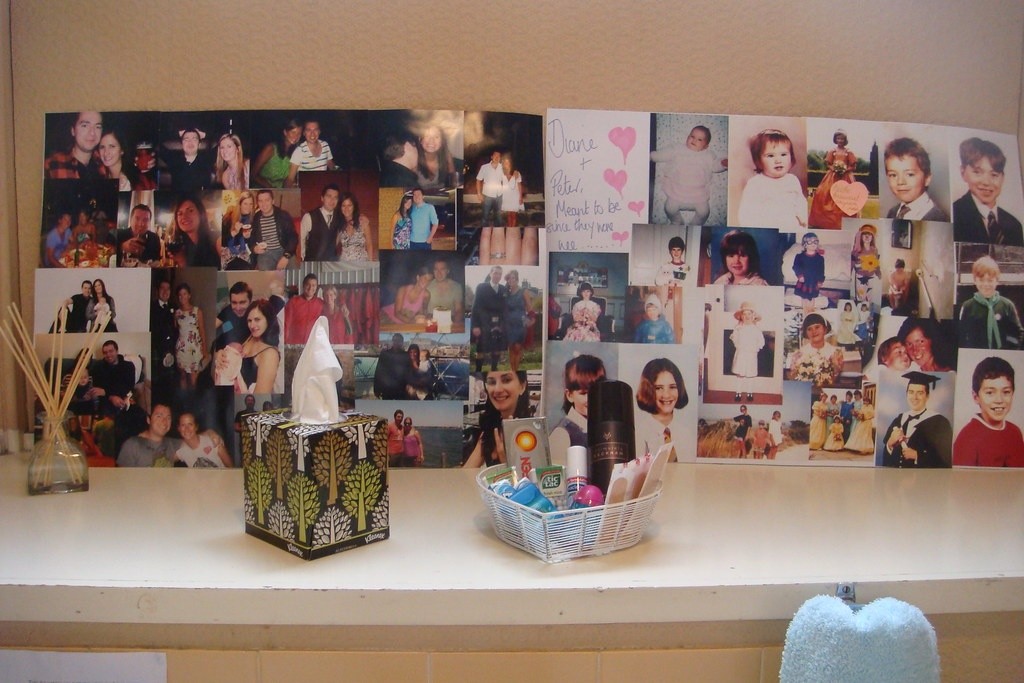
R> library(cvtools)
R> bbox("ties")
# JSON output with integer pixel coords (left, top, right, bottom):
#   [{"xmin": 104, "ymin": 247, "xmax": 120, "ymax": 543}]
[
  {"xmin": 973, "ymin": 291, "xmax": 1001, "ymax": 350},
  {"xmin": 327, "ymin": 214, "xmax": 333, "ymax": 229},
  {"xmin": 987, "ymin": 209, "xmax": 1005, "ymax": 245},
  {"xmin": 897, "ymin": 205, "xmax": 909, "ymax": 220},
  {"xmin": 163, "ymin": 304, "xmax": 168, "ymax": 310},
  {"xmin": 663, "ymin": 428, "xmax": 678, "ymax": 462}
]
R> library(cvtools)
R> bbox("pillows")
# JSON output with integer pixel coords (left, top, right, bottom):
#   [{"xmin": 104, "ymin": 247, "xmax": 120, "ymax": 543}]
[{"xmin": 124, "ymin": 354, "xmax": 142, "ymax": 384}]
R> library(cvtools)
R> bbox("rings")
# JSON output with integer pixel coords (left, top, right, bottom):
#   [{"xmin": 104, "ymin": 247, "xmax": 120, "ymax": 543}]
[
  {"xmin": 490, "ymin": 252, "xmax": 506, "ymax": 259},
  {"xmin": 136, "ymin": 250, "xmax": 138, "ymax": 253},
  {"xmin": 93, "ymin": 391, "xmax": 96, "ymax": 392}
]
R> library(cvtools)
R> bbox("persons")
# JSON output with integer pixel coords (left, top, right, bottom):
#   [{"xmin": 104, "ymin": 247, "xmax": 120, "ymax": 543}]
[
  {"xmin": 31, "ymin": 107, "xmax": 546, "ymax": 470},
  {"xmin": 649, "ymin": 113, "xmax": 1024, "ymax": 248},
  {"xmin": 547, "ymin": 228, "xmax": 1024, "ymax": 469}
]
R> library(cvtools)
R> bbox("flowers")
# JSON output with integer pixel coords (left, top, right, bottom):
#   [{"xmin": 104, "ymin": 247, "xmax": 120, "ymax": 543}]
[
  {"xmin": 821, "ymin": 393, "xmax": 825, "ymax": 395},
  {"xmin": 831, "ymin": 409, "xmax": 839, "ymax": 415},
  {"xmin": 820, "ymin": 407, "xmax": 826, "ymax": 418},
  {"xmin": 832, "ymin": 160, "xmax": 847, "ymax": 174},
  {"xmin": 858, "ymin": 254, "xmax": 879, "ymax": 272},
  {"xmin": 851, "ymin": 408, "xmax": 864, "ymax": 420},
  {"xmin": 788, "ymin": 349, "xmax": 844, "ymax": 388}
]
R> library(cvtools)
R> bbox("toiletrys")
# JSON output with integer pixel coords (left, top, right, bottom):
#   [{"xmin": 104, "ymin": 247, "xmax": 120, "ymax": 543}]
[
  {"xmin": 565, "ymin": 445, "xmax": 587, "ymax": 509},
  {"xmin": 587, "ymin": 379, "xmax": 637, "ymax": 506},
  {"xmin": 566, "ymin": 485, "xmax": 604, "ymax": 546}
]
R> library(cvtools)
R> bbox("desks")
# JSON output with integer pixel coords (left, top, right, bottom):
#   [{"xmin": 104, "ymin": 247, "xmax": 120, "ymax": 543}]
[
  {"xmin": 379, "ymin": 323, "xmax": 465, "ymax": 333},
  {"xmin": 784, "ymin": 351, "xmax": 862, "ymax": 381}
]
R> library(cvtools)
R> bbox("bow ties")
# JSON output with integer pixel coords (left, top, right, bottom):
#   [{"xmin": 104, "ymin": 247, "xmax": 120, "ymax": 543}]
[{"xmin": 909, "ymin": 408, "xmax": 927, "ymax": 420}]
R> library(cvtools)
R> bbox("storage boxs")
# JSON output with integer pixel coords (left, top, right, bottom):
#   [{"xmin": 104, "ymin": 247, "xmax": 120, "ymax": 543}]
[
  {"xmin": 527, "ymin": 464, "xmax": 568, "ymax": 511},
  {"xmin": 482, "ymin": 465, "xmax": 518, "ymax": 493}
]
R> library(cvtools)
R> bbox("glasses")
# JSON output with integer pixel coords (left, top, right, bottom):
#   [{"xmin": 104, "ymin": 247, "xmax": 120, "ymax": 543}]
[{"xmin": 802, "ymin": 239, "xmax": 820, "ymax": 246}]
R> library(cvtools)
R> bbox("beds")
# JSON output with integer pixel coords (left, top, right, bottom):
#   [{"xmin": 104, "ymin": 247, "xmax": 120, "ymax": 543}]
[{"xmin": 776, "ymin": 233, "xmax": 855, "ymax": 300}]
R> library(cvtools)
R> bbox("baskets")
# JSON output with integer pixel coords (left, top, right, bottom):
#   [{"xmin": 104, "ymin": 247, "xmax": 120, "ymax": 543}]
[{"xmin": 476, "ymin": 461, "xmax": 665, "ymax": 564}]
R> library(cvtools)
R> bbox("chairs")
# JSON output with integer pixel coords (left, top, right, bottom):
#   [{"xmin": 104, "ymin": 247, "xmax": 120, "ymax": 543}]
[
  {"xmin": 555, "ymin": 296, "xmax": 616, "ymax": 342},
  {"xmin": 837, "ymin": 299, "xmax": 870, "ymax": 331}
]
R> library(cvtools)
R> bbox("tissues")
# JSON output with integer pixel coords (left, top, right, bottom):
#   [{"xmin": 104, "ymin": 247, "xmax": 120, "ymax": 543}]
[{"xmin": 239, "ymin": 315, "xmax": 390, "ymax": 562}]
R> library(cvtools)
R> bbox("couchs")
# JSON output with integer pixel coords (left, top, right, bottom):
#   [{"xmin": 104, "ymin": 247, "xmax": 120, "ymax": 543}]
[{"xmin": 34, "ymin": 358, "xmax": 147, "ymax": 426}]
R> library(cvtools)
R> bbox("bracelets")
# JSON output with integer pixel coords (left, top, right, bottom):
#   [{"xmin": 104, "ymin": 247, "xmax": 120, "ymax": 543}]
[{"xmin": 478, "ymin": 193, "xmax": 481, "ymax": 196}]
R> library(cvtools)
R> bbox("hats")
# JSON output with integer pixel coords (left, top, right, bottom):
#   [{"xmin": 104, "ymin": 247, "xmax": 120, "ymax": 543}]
[
  {"xmin": 901, "ymin": 369, "xmax": 940, "ymax": 390},
  {"xmin": 734, "ymin": 301, "xmax": 761, "ymax": 323},
  {"xmin": 645, "ymin": 293, "xmax": 661, "ymax": 311}
]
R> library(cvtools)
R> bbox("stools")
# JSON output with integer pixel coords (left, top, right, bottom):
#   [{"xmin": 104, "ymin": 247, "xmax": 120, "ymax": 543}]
[{"xmin": 840, "ymin": 372, "xmax": 866, "ymax": 388}]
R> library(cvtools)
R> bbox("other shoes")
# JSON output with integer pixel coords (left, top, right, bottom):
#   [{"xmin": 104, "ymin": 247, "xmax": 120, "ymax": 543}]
[
  {"xmin": 747, "ymin": 393, "xmax": 753, "ymax": 402},
  {"xmin": 736, "ymin": 393, "xmax": 742, "ymax": 401}
]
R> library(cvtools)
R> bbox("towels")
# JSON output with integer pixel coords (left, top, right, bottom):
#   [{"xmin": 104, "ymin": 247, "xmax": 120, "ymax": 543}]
[{"xmin": 778, "ymin": 593, "xmax": 941, "ymax": 683}]
